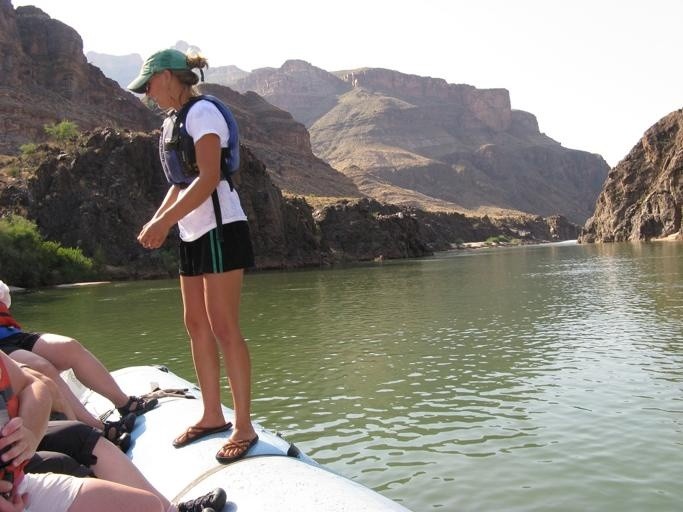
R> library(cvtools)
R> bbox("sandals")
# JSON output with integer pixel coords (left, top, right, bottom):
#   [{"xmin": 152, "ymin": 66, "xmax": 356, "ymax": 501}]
[
  {"xmin": 117, "ymin": 396, "xmax": 158, "ymax": 417},
  {"xmin": 103, "ymin": 414, "xmax": 135, "ymax": 453}
]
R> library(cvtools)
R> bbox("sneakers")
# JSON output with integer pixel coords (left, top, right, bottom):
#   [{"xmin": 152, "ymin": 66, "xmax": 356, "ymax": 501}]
[{"xmin": 178, "ymin": 488, "xmax": 227, "ymax": 512}]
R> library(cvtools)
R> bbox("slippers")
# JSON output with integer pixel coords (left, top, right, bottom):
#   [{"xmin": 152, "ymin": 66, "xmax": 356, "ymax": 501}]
[
  {"xmin": 215, "ymin": 433, "xmax": 258, "ymax": 462},
  {"xmin": 172, "ymin": 421, "xmax": 233, "ymax": 448}
]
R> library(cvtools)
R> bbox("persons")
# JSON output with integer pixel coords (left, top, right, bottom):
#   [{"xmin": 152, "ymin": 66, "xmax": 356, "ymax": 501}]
[
  {"xmin": 0, "ymin": 279, "xmax": 227, "ymax": 512},
  {"xmin": 128, "ymin": 49, "xmax": 257, "ymax": 463}
]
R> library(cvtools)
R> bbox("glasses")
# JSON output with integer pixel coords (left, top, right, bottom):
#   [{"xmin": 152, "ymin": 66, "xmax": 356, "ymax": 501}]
[{"xmin": 143, "ymin": 83, "xmax": 151, "ymax": 92}]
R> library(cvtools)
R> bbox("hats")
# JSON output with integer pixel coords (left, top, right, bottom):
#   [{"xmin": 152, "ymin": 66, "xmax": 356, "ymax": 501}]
[{"xmin": 126, "ymin": 48, "xmax": 190, "ymax": 93}]
